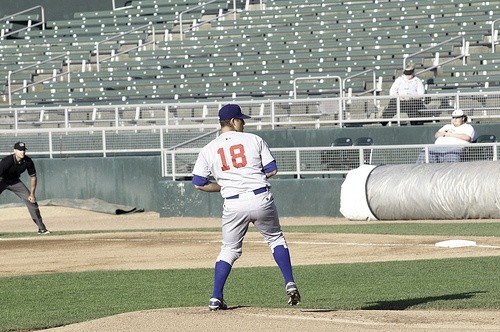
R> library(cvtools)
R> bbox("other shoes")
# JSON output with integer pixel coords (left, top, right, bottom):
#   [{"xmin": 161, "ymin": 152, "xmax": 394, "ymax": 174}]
[
  {"xmin": 209, "ymin": 298, "xmax": 227, "ymax": 311},
  {"xmin": 38, "ymin": 230, "xmax": 50, "ymax": 235},
  {"xmin": 286, "ymin": 282, "xmax": 301, "ymax": 306}
]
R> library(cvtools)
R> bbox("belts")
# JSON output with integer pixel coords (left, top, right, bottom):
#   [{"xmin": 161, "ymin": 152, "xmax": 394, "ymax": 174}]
[{"xmin": 226, "ymin": 187, "xmax": 267, "ymax": 199}]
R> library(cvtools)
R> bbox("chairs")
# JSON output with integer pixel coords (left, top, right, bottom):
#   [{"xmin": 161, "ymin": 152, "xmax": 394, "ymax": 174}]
[
  {"xmin": 461, "ymin": 135, "xmax": 496, "ymax": 161},
  {"xmin": 321, "ymin": 137, "xmax": 373, "ymax": 178}
]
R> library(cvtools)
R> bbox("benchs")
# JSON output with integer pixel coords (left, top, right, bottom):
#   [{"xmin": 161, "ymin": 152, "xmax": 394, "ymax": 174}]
[{"xmin": 0, "ymin": 0, "xmax": 500, "ymax": 133}]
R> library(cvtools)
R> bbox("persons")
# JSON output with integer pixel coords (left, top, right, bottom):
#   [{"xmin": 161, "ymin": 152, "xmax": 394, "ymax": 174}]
[
  {"xmin": 416, "ymin": 108, "xmax": 475, "ymax": 163},
  {"xmin": 0, "ymin": 141, "xmax": 51, "ymax": 235},
  {"xmin": 191, "ymin": 104, "xmax": 301, "ymax": 310},
  {"xmin": 378, "ymin": 64, "xmax": 426, "ymax": 126}
]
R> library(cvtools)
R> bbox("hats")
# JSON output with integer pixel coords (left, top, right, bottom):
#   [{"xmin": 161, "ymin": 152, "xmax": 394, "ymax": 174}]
[
  {"xmin": 14, "ymin": 142, "xmax": 27, "ymax": 150},
  {"xmin": 452, "ymin": 109, "xmax": 464, "ymax": 117},
  {"xmin": 218, "ymin": 104, "xmax": 251, "ymax": 120},
  {"xmin": 403, "ymin": 68, "xmax": 414, "ymax": 75}
]
